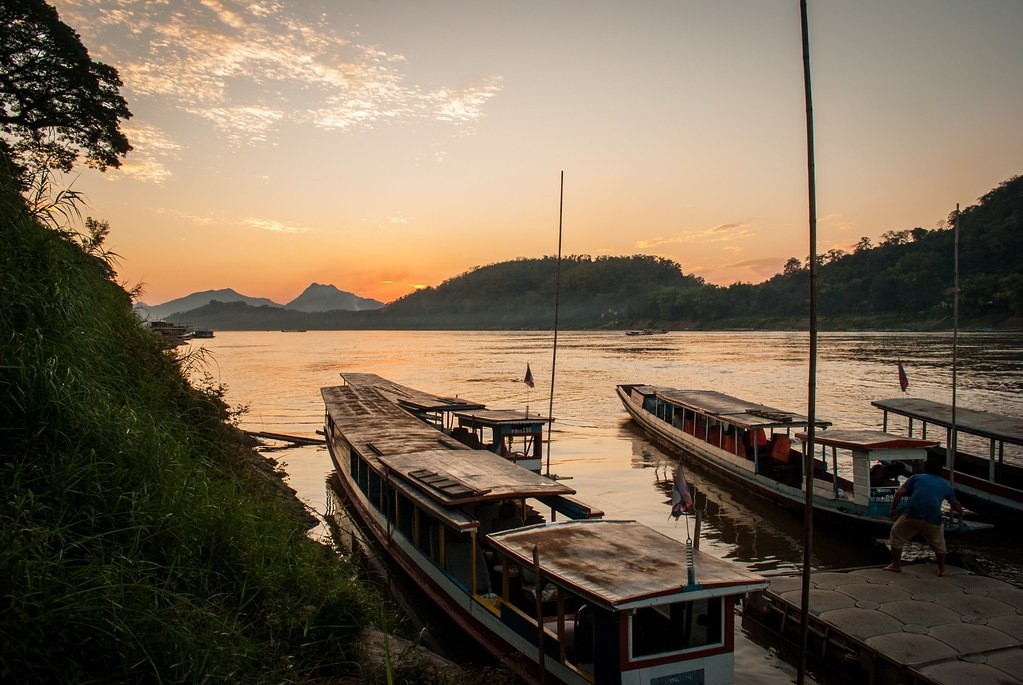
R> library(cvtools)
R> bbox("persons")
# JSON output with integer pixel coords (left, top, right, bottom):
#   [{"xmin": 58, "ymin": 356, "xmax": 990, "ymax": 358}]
[
  {"xmin": 883, "ymin": 458, "xmax": 962, "ymax": 575},
  {"xmin": 524, "ymin": 366, "xmax": 534, "ymax": 388}
]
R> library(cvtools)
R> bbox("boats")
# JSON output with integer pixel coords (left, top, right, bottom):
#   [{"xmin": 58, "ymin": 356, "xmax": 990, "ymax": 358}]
[
  {"xmin": 193, "ymin": 329, "xmax": 217, "ymax": 339},
  {"xmin": 282, "ymin": 329, "xmax": 308, "ymax": 332},
  {"xmin": 625, "ymin": 329, "xmax": 669, "ymax": 336},
  {"xmin": 869, "ymin": 360, "xmax": 1022, "ymax": 524},
  {"xmin": 321, "ymin": 0, "xmax": 808, "ymax": 685},
  {"xmin": 338, "ymin": 171, "xmax": 574, "ymax": 487},
  {"xmin": 614, "ymin": 202, "xmax": 990, "ymax": 536}
]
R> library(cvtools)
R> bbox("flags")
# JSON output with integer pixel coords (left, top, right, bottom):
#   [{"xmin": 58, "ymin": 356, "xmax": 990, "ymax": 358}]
[
  {"xmin": 670, "ymin": 458, "xmax": 691, "ymax": 517},
  {"xmin": 898, "ymin": 356, "xmax": 909, "ymax": 392}
]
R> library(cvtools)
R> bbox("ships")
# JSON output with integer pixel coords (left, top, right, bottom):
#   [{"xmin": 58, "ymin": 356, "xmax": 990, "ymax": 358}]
[{"xmin": 149, "ymin": 320, "xmax": 198, "ymax": 338}]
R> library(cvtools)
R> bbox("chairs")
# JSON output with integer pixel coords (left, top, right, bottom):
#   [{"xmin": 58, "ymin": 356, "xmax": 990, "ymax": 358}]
[
  {"xmin": 387, "ymin": 484, "xmax": 663, "ymax": 663},
  {"xmin": 771, "ymin": 435, "xmax": 791, "ymax": 461},
  {"xmin": 750, "ymin": 427, "xmax": 767, "ymax": 448},
  {"xmin": 645, "ymin": 397, "xmax": 745, "ymax": 452},
  {"xmin": 725, "ymin": 435, "xmax": 746, "ymax": 458},
  {"xmin": 461, "ymin": 432, "xmax": 480, "ymax": 449},
  {"xmin": 452, "ymin": 427, "xmax": 468, "ymax": 442}
]
